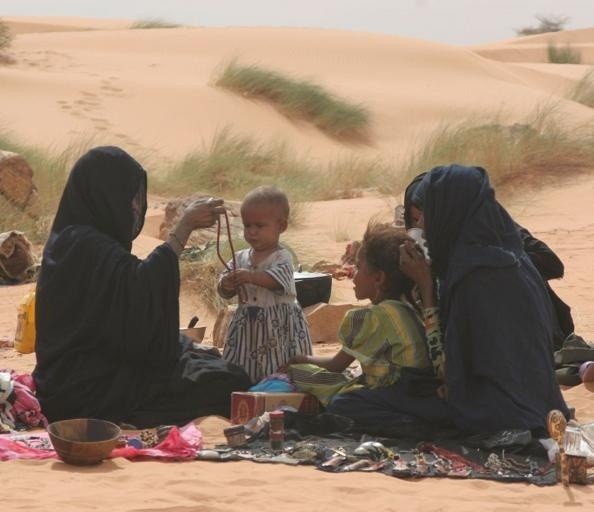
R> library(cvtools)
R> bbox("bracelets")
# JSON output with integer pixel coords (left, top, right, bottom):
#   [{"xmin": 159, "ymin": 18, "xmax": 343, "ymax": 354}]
[{"xmin": 166, "ymin": 230, "xmax": 186, "ymax": 254}]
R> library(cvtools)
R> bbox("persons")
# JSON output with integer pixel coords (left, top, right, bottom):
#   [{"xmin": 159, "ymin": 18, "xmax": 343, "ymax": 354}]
[
  {"xmin": 214, "ymin": 185, "xmax": 313, "ymax": 388},
  {"xmin": 30, "ymin": 145, "xmax": 251, "ymax": 431}
]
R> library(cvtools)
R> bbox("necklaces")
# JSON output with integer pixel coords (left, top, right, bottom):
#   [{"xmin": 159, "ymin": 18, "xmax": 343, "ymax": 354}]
[{"xmin": 214, "ymin": 208, "xmax": 252, "ymax": 304}]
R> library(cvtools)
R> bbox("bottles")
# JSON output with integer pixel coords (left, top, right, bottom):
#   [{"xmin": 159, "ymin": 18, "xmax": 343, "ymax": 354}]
[{"xmin": 268, "ymin": 411, "xmax": 285, "ymax": 455}]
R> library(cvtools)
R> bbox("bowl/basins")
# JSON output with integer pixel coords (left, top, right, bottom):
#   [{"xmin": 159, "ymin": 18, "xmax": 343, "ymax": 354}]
[{"xmin": 45, "ymin": 417, "xmax": 123, "ymax": 467}]
